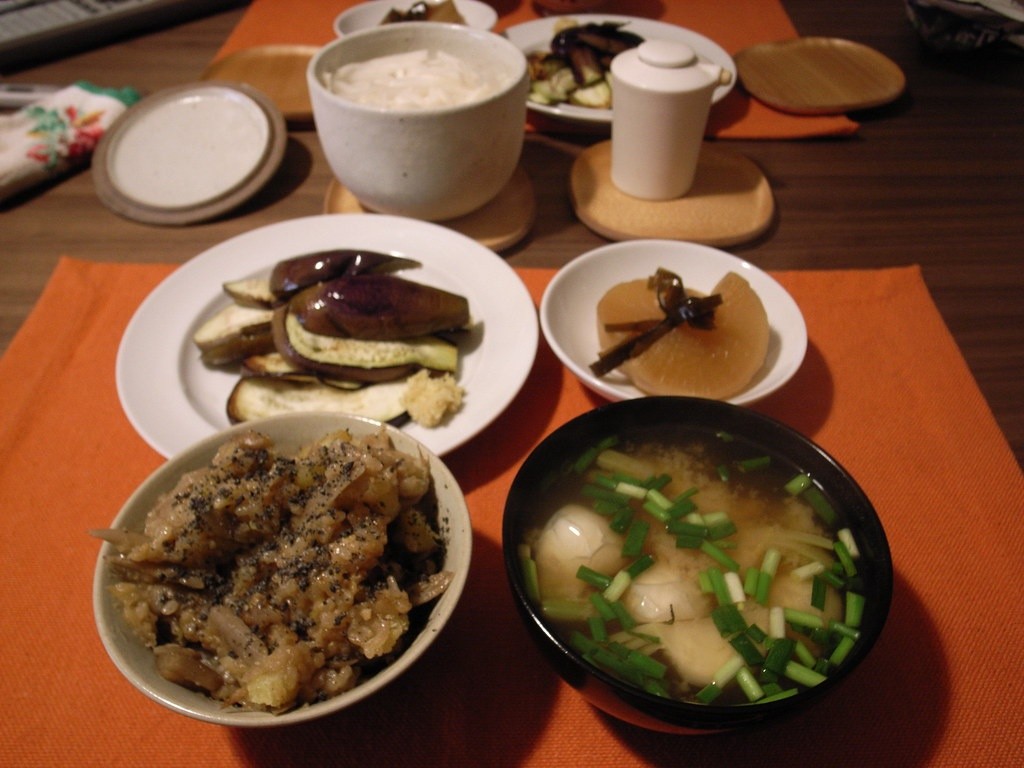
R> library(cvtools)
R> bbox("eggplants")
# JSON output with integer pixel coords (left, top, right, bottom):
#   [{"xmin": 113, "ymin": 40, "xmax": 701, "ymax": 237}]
[
  {"xmin": 527, "ymin": 20, "xmax": 731, "ymax": 108},
  {"xmin": 193, "ymin": 249, "xmax": 471, "ymax": 429},
  {"xmin": 378, "ymin": 0, "xmax": 467, "ymax": 25}
]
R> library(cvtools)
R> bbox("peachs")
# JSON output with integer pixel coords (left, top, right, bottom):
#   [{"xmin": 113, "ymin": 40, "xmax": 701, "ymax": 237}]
[{"xmin": 597, "ymin": 271, "xmax": 770, "ymax": 397}]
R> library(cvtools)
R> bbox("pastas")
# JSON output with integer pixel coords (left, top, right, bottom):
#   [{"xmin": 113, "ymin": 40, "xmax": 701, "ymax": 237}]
[{"xmin": 323, "ymin": 49, "xmax": 491, "ymax": 107}]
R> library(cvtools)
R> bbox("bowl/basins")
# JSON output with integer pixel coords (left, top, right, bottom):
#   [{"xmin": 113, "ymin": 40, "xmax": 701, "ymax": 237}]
[
  {"xmin": 93, "ymin": 412, "xmax": 473, "ymax": 728},
  {"xmin": 501, "ymin": 396, "xmax": 893, "ymax": 736},
  {"xmin": 306, "ymin": 22, "xmax": 531, "ymax": 221}
]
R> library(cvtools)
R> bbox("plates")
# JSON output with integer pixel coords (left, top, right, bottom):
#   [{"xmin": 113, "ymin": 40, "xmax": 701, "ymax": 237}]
[
  {"xmin": 541, "ymin": 239, "xmax": 809, "ymax": 409},
  {"xmin": 501, "ymin": 14, "xmax": 738, "ymax": 123},
  {"xmin": 115, "ymin": 212, "xmax": 539, "ymax": 459},
  {"xmin": 335, "ymin": 0, "xmax": 498, "ymax": 38}
]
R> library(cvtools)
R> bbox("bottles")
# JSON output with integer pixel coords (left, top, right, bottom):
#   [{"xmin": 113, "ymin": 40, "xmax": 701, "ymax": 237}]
[{"xmin": 611, "ymin": 40, "xmax": 724, "ymax": 199}]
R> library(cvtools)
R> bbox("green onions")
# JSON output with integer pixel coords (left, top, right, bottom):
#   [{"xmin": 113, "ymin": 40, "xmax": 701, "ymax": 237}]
[{"xmin": 518, "ymin": 433, "xmax": 866, "ymax": 702}]
